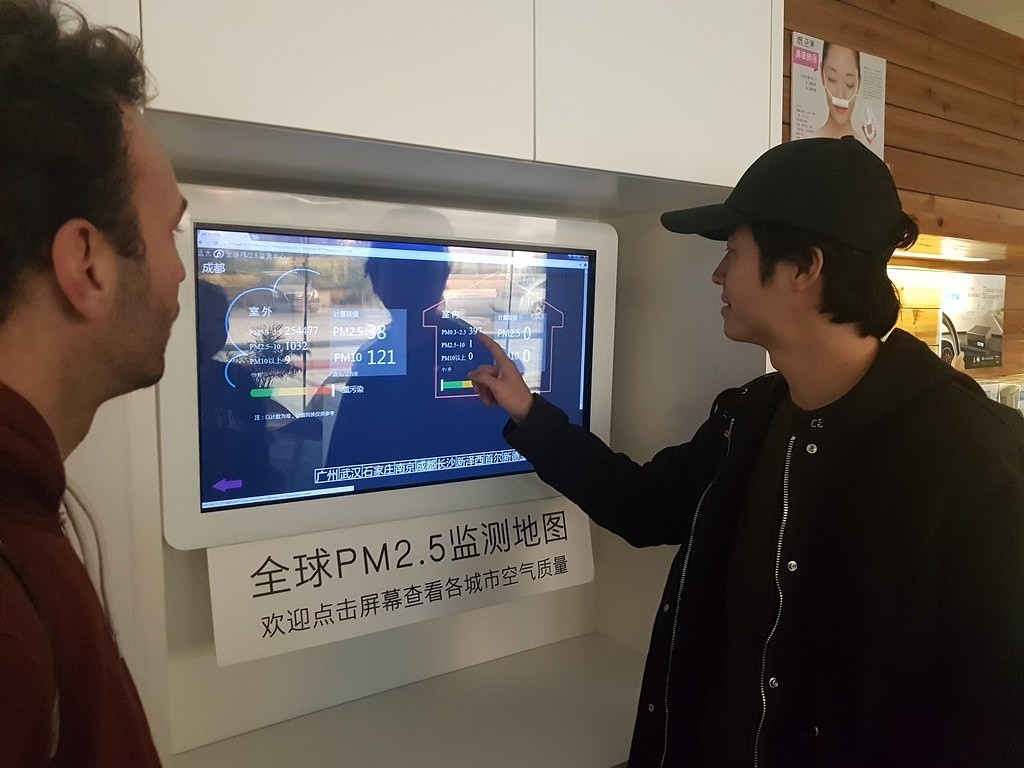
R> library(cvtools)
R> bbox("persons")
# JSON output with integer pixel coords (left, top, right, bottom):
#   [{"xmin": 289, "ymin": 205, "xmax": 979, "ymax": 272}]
[
  {"xmin": 195, "ymin": 279, "xmax": 269, "ymax": 503},
  {"xmin": 0, "ymin": 0, "xmax": 187, "ymax": 768},
  {"xmin": 315, "ymin": 209, "xmax": 525, "ymax": 492},
  {"xmin": 469, "ymin": 134, "xmax": 1024, "ymax": 768},
  {"xmin": 800, "ymin": 42, "xmax": 861, "ymax": 139}
]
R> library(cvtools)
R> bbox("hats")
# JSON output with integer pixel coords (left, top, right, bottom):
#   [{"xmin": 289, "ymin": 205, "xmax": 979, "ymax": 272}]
[{"xmin": 659, "ymin": 135, "xmax": 902, "ymax": 252}]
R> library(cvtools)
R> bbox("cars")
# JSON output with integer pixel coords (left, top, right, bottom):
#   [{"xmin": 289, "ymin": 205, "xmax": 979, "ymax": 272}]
[
  {"xmin": 496, "ymin": 273, "xmax": 547, "ymax": 321},
  {"xmin": 255, "ymin": 270, "xmax": 320, "ymax": 316},
  {"xmin": 443, "ymin": 271, "xmax": 506, "ymax": 320}
]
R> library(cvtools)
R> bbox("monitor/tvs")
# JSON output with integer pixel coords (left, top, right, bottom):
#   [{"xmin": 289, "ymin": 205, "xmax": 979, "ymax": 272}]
[{"xmin": 152, "ymin": 186, "xmax": 619, "ymax": 552}]
